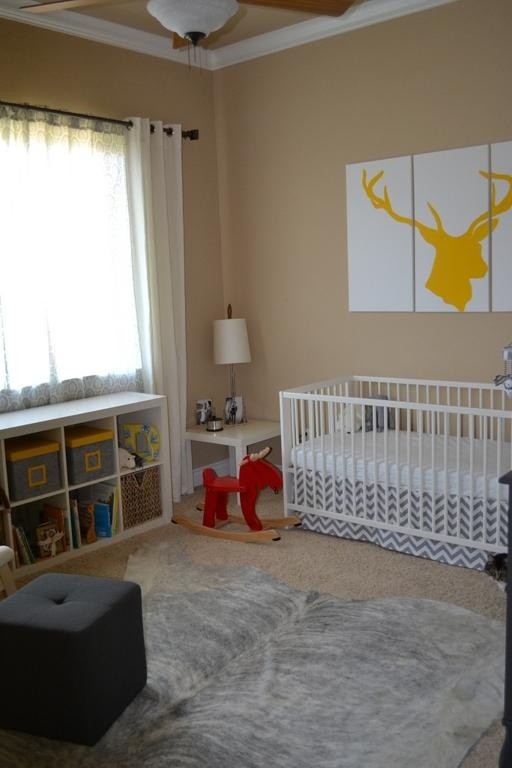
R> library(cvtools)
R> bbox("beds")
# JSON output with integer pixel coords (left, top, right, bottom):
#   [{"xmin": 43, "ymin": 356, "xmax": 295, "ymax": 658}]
[{"xmin": 278, "ymin": 371, "xmax": 512, "ymax": 591}]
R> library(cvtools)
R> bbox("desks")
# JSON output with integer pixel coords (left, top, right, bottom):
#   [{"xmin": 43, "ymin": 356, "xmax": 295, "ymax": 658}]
[{"xmin": 184, "ymin": 418, "xmax": 281, "ymax": 505}]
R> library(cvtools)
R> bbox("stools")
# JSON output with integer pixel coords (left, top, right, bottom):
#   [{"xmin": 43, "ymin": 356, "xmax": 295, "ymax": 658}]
[{"xmin": 0, "ymin": 571, "xmax": 147, "ymax": 747}]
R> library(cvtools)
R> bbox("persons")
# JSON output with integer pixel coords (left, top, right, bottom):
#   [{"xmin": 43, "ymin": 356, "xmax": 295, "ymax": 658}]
[
  {"xmin": 196, "ymin": 403, "xmax": 208, "ymax": 425},
  {"xmin": 230, "ymin": 402, "xmax": 238, "ymax": 424},
  {"xmin": 206, "ymin": 400, "xmax": 212, "ymax": 419}
]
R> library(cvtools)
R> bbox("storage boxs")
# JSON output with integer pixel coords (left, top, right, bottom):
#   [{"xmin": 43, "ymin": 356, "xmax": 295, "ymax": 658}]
[{"xmin": 7, "ymin": 424, "xmax": 114, "ymax": 502}]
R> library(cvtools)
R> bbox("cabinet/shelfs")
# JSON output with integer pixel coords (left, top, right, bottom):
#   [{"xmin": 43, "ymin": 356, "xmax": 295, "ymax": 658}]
[{"xmin": 0, "ymin": 389, "xmax": 174, "ymax": 584}]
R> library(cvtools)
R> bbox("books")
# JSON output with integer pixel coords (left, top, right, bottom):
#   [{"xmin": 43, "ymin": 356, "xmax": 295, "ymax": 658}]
[{"xmin": 12, "ymin": 481, "xmax": 119, "ymax": 568}]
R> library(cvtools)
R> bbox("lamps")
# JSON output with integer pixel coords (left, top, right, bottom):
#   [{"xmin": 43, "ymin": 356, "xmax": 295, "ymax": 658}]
[
  {"xmin": 145, "ymin": 0, "xmax": 239, "ymax": 48},
  {"xmin": 211, "ymin": 304, "xmax": 252, "ymax": 426}
]
